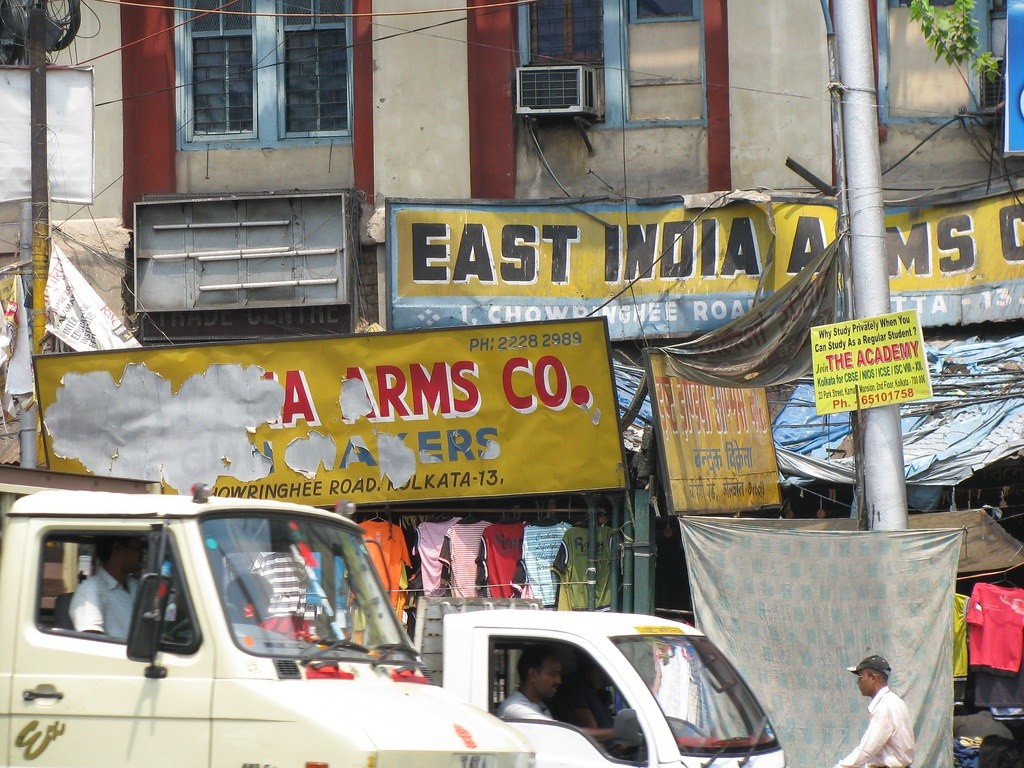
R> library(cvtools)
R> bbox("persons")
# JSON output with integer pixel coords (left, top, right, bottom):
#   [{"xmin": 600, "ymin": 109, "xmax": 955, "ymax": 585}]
[
  {"xmin": 68, "ymin": 535, "xmax": 145, "ymax": 639},
  {"xmin": 832, "ymin": 655, "xmax": 917, "ymax": 768},
  {"xmin": 497, "ymin": 642, "xmax": 638, "ymax": 759}
]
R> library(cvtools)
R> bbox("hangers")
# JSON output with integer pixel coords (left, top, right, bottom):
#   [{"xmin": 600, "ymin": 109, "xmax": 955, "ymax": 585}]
[{"xmin": 364, "ymin": 500, "xmax": 607, "ymax": 525}]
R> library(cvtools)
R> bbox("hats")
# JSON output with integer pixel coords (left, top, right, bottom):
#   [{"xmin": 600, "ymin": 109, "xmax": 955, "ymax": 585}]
[{"xmin": 846, "ymin": 655, "xmax": 891, "ymax": 677}]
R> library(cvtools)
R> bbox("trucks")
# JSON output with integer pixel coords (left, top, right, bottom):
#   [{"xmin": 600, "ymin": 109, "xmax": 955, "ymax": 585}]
[
  {"xmin": 1, "ymin": 463, "xmax": 536, "ymax": 768},
  {"xmin": 403, "ymin": 597, "xmax": 790, "ymax": 767}
]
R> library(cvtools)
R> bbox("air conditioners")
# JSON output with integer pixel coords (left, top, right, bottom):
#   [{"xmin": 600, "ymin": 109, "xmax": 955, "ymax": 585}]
[{"xmin": 514, "ymin": 63, "xmax": 599, "ymax": 114}]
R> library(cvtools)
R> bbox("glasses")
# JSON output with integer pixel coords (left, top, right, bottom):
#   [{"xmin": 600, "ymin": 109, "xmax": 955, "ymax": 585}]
[{"xmin": 858, "ymin": 674, "xmax": 877, "ymax": 681}]
